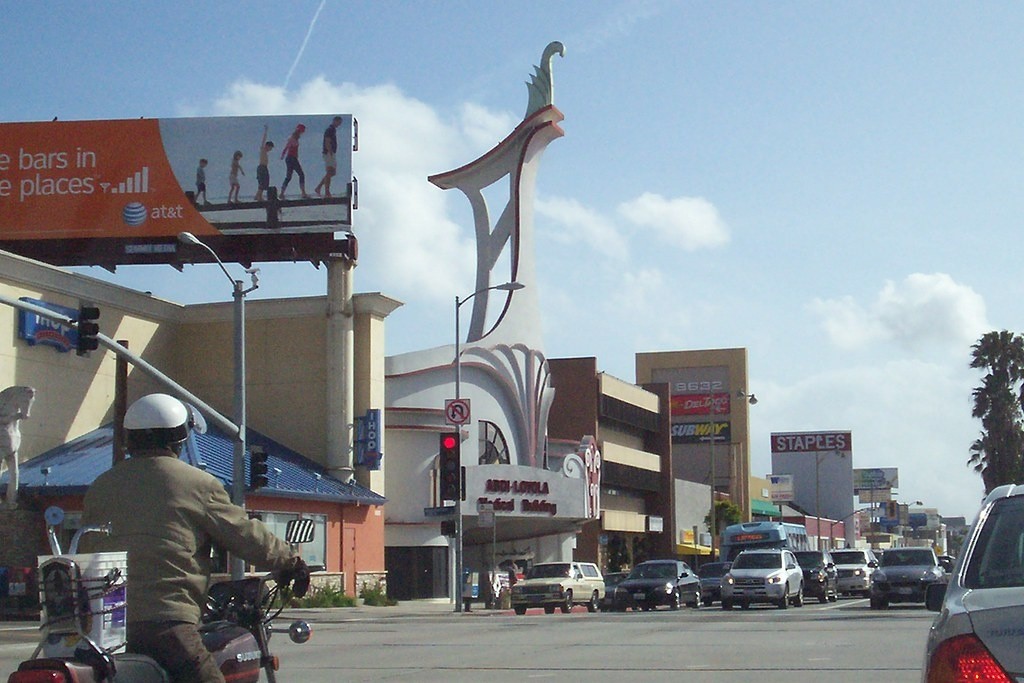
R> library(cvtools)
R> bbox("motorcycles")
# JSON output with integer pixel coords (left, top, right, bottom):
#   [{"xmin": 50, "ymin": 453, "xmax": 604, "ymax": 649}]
[{"xmin": 6, "ymin": 518, "xmax": 317, "ymax": 683}]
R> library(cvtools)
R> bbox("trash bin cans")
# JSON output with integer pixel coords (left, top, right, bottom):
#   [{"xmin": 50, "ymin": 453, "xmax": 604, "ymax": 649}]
[
  {"xmin": 1, "ymin": 566, "xmax": 40, "ymax": 610},
  {"xmin": 501, "ymin": 587, "xmax": 513, "ymax": 609},
  {"xmin": 464, "ymin": 571, "xmax": 480, "ymax": 597}
]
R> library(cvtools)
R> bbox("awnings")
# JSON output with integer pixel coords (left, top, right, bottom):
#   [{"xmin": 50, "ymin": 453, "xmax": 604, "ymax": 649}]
[
  {"xmin": 677, "ymin": 544, "xmax": 720, "ymax": 555},
  {"xmin": 750, "ymin": 499, "xmax": 781, "ymax": 517}
]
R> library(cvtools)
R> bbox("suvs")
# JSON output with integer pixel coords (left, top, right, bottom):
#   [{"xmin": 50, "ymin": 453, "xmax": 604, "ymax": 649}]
[
  {"xmin": 826, "ymin": 549, "xmax": 880, "ymax": 599},
  {"xmin": 792, "ymin": 550, "xmax": 838, "ymax": 604},
  {"xmin": 922, "ymin": 484, "xmax": 1023, "ymax": 683},
  {"xmin": 719, "ymin": 547, "xmax": 804, "ymax": 610},
  {"xmin": 509, "ymin": 561, "xmax": 606, "ymax": 616},
  {"xmin": 866, "ymin": 546, "xmax": 949, "ymax": 609}
]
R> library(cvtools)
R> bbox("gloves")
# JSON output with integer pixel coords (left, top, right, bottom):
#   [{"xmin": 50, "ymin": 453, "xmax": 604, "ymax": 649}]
[{"xmin": 278, "ymin": 559, "xmax": 310, "ymax": 598}]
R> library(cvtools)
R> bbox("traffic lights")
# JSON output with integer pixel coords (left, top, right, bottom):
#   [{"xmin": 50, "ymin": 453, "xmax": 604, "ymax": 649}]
[
  {"xmin": 438, "ymin": 432, "xmax": 460, "ymax": 501},
  {"xmin": 440, "ymin": 519, "xmax": 456, "ymax": 536},
  {"xmin": 75, "ymin": 302, "xmax": 101, "ymax": 356},
  {"xmin": 249, "ymin": 443, "xmax": 270, "ymax": 490}
]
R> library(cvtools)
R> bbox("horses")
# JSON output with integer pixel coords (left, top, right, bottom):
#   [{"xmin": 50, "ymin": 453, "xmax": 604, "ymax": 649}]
[{"xmin": 0, "ymin": 386, "xmax": 37, "ymax": 504}]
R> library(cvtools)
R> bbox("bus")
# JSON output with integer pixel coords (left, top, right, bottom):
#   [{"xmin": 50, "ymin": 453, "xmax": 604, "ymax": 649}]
[{"xmin": 718, "ymin": 519, "xmax": 811, "ymax": 561}]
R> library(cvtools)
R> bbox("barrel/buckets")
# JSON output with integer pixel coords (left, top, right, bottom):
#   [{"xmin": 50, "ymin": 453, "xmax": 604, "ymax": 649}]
[{"xmin": 38, "ymin": 551, "xmax": 128, "ymax": 658}]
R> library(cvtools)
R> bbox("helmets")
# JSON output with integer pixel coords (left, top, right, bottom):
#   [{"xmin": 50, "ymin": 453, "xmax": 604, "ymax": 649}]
[{"xmin": 124, "ymin": 393, "xmax": 189, "ymax": 444}]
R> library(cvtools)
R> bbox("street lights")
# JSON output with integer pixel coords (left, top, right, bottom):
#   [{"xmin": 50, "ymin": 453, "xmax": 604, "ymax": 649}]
[
  {"xmin": 903, "ymin": 500, "xmax": 925, "ymax": 547},
  {"xmin": 708, "ymin": 378, "xmax": 758, "ymax": 563},
  {"xmin": 175, "ymin": 230, "xmax": 247, "ymax": 581},
  {"xmin": 454, "ymin": 279, "xmax": 528, "ymax": 613},
  {"xmin": 814, "ymin": 444, "xmax": 847, "ymax": 551}
]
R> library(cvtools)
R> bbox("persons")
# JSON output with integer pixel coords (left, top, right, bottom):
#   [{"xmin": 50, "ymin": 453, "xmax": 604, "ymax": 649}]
[
  {"xmin": 254, "ymin": 124, "xmax": 274, "ymax": 201},
  {"xmin": 193, "ymin": 159, "xmax": 209, "ymax": 205},
  {"xmin": 76, "ymin": 393, "xmax": 311, "ymax": 683},
  {"xmin": 509, "ymin": 562, "xmax": 518, "ymax": 591},
  {"xmin": 480, "ymin": 564, "xmax": 492, "ymax": 609},
  {"xmin": 316, "ymin": 116, "xmax": 343, "ymax": 198},
  {"xmin": 228, "ymin": 150, "xmax": 246, "ymax": 203},
  {"xmin": 278, "ymin": 124, "xmax": 311, "ymax": 200}
]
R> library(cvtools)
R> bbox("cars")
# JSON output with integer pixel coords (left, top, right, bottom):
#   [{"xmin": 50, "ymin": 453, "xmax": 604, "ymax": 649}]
[
  {"xmin": 617, "ymin": 558, "xmax": 703, "ymax": 611},
  {"xmin": 938, "ymin": 555, "xmax": 955, "ymax": 582},
  {"xmin": 596, "ymin": 572, "xmax": 631, "ymax": 613},
  {"xmin": 694, "ymin": 561, "xmax": 732, "ymax": 607}
]
